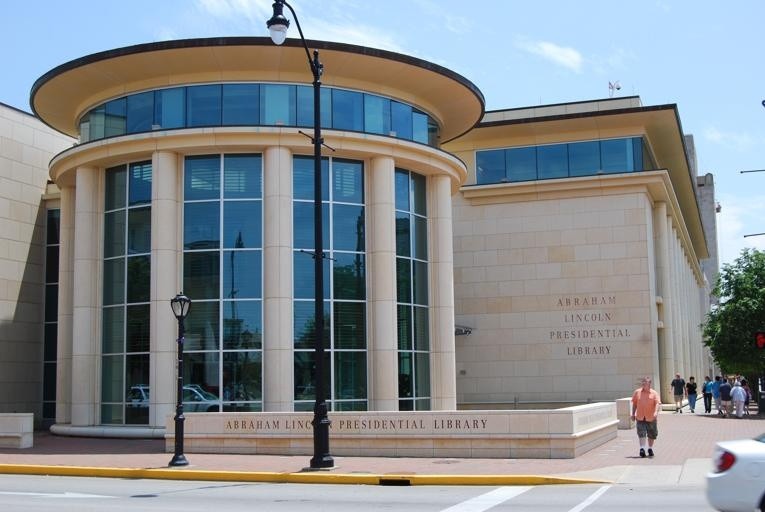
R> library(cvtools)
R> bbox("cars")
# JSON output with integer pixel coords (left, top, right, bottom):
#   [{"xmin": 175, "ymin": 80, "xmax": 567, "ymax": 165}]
[
  {"xmin": 128, "ymin": 384, "xmax": 221, "ymax": 415},
  {"xmin": 705, "ymin": 431, "xmax": 765, "ymax": 512}
]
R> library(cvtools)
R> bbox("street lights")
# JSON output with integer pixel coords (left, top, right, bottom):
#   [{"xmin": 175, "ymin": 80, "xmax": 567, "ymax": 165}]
[
  {"xmin": 265, "ymin": 0, "xmax": 336, "ymax": 469},
  {"xmin": 170, "ymin": 290, "xmax": 192, "ymax": 465}
]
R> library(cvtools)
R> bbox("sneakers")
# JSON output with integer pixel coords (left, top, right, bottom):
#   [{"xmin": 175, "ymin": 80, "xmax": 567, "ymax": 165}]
[
  {"xmin": 648, "ymin": 448, "xmax": 654, "ymax": 456},
  {"xmin": 640, "ymin": 448, "xmax": 646, "ymax": 457}
]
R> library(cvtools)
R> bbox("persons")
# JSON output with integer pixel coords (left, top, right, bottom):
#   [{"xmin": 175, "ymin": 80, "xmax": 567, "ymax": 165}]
[
  {"xmin": 669, "ymin": 373, "xmax": 687, "ymax": 415},
  {"xmin": 685, "ymin": 376, "xmax": 698, "ymax": 413},
  {"xmin": 630, "ymin": 375, "xmax": 662, "ymax": 457},
  {"xmin": 703, "ymin": 372, "xmax": 752, "ymax": 418}
]
